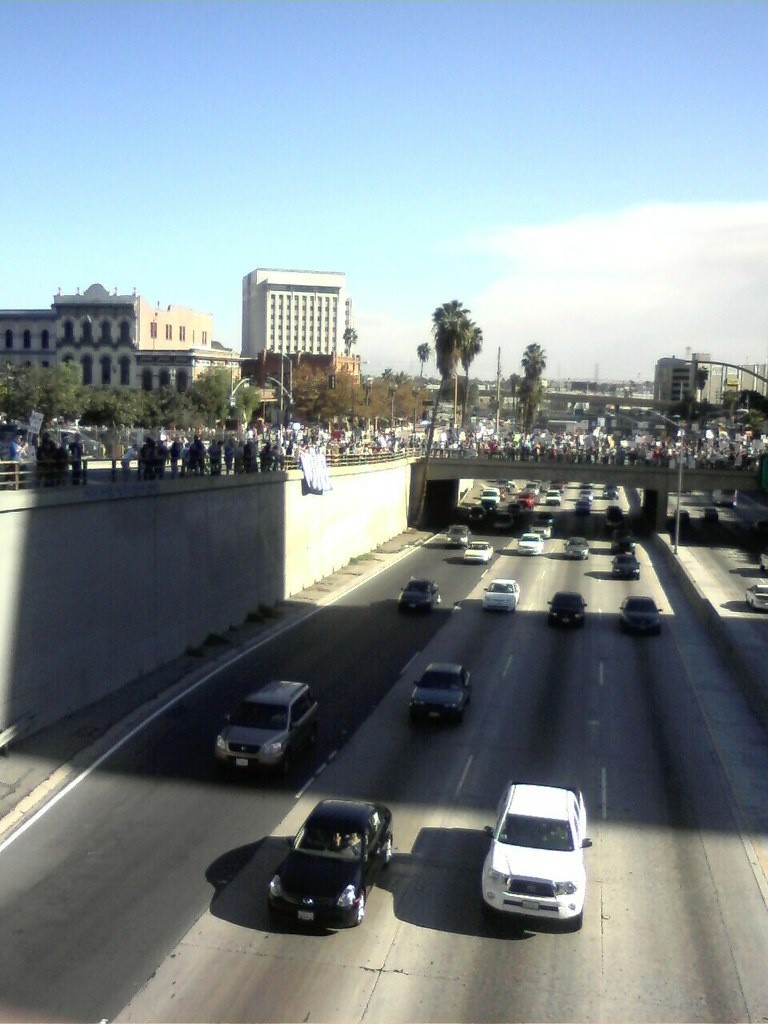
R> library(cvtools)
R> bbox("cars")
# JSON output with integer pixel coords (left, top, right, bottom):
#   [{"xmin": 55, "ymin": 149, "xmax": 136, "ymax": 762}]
[
  {"xmin": 266, "ymin": 797, "xmax": 393, "ymax": 929},
  {"xmin": 563, "ymin": 536, "xmax": 589, "ymax": 560},
  {"xmin": 751, "ymin": 519, "xmax": 768, "ymax": 533},
  {"xmin": 517, "ymin": 532, "xmax": 543, "ymax": 557},
  {"xmin": 674, "ymin": 509, "xmax": 689, "ymax": 527},
  {"xmin": 529, "ymin": 522, "xmax": 552, "ymax": 540},
  {"xmin": 700, "ymin": 507, "xmax": 718, "ymax": 523},
  {"xmin": 494, "ymin": 512, "xmax": 515, "ymax": 528},
  {"xmin": 398, "ymin": 578, "xmax": 440, "ymax": 616},
  {"xmin": 480, "ymin": 480, "xmax": 625, "ymax": 531},
  {"xmin": 744, "ymin": 585, "xmax": 768, "ymax": 611},
  {"xmin": 467, "ymin": 505, "xmax": 487, "ymax": 523},
  {"xmin": 408, "ymin": 662, "xmax": 473, "ymax": 730},
  {"xmin": 445, "ymin": 525, "xmax": 472, "ymax": 548},
  {"xmin": 757, "ymin": 548, "xmax": 768, "ymax": 575},
  {"xmin": 546, "ymin": 591, "xmax": 588, "ymax": 628},
  {"xmin": 462, "ymin": 540, "xmax": 494, "ymax": 565},
  {"xmin": 482, "ymin": 578, "xmax": 520, "ymax": 612},
  {"xmin": 611, "ymin": 553, "xmax": 640, "ymax": 581},
  {"xmin": 618, "ymin": 596, "xmax": 663, "ymax": 635}
]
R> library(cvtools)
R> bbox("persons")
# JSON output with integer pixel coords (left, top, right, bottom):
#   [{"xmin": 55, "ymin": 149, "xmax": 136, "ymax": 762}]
[
  {"xmin": 10, "ymin": 426, "xmax": 768, "ymax": 488},
  {"xmin": 330, "ymin": 833, "xmax": 361, "ymax": 856}
]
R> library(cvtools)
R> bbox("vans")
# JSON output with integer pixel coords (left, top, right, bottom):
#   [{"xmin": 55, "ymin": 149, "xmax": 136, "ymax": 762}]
[{"xmin": 612, "ymin": 528, "xmax": 637, "ymax": 554}]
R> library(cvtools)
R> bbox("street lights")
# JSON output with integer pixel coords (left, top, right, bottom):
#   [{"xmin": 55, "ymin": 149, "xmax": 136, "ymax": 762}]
[{"xmin": 282, "ymin": 352, "xmax": 292, "ymax": 402}]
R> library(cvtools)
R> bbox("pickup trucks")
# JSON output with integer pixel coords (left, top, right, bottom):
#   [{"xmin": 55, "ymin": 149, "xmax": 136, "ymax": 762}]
[{"xmin": 480, "ymin": 782, "xmax": 594, "ymax": 931}]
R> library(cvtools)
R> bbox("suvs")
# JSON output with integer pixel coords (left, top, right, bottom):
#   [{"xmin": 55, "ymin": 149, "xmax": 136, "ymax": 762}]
[{"xmin": 212, "ymin": 681, "xmax": 320, "ymax": 770}]
